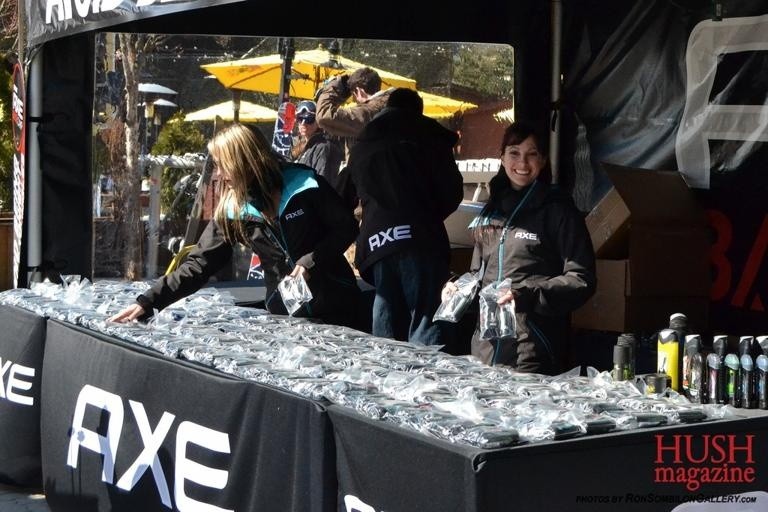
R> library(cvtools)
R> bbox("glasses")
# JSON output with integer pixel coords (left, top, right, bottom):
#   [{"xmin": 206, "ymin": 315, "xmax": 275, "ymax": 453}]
[{"xmin": 295, "ymin": 115, "xmax": 314, "ymax": 124}]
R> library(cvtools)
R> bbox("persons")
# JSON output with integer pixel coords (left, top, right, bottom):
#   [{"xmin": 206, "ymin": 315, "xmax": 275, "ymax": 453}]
[
  {"xmin": 104, "ymin": 68, "xmax": 465, "ymax": 353},
  {"xmin": 440, "ymin": 119, "xmax": 599, "ymax": 376}
]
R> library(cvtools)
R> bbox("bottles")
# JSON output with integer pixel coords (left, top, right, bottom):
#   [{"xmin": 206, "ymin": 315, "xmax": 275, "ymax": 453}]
[{"xmin": 612, "ymin": 332, "xmax": 637, "ymax": 380}]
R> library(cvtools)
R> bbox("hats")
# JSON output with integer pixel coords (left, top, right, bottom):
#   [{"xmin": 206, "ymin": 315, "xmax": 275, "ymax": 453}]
[{"xmin": 294, "ymin": 100, "xmax": 318, "ymax": 114}]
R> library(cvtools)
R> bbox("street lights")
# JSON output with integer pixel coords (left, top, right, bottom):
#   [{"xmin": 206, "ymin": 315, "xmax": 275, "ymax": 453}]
[{"xmin": 137, "ymin": 81, "xmax": 178, "ymax": 154}]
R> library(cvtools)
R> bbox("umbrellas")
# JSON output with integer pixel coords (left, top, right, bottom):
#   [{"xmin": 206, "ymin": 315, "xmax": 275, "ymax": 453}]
[{"xmin": 199, "ymin": 41, "xmax": 417, "ymax": 104}]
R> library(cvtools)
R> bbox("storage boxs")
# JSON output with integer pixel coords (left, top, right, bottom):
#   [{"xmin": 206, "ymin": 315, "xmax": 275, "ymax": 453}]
[{"xmin": 571, "ymin": 163, "xmax": 710, "ymax": 333}]
